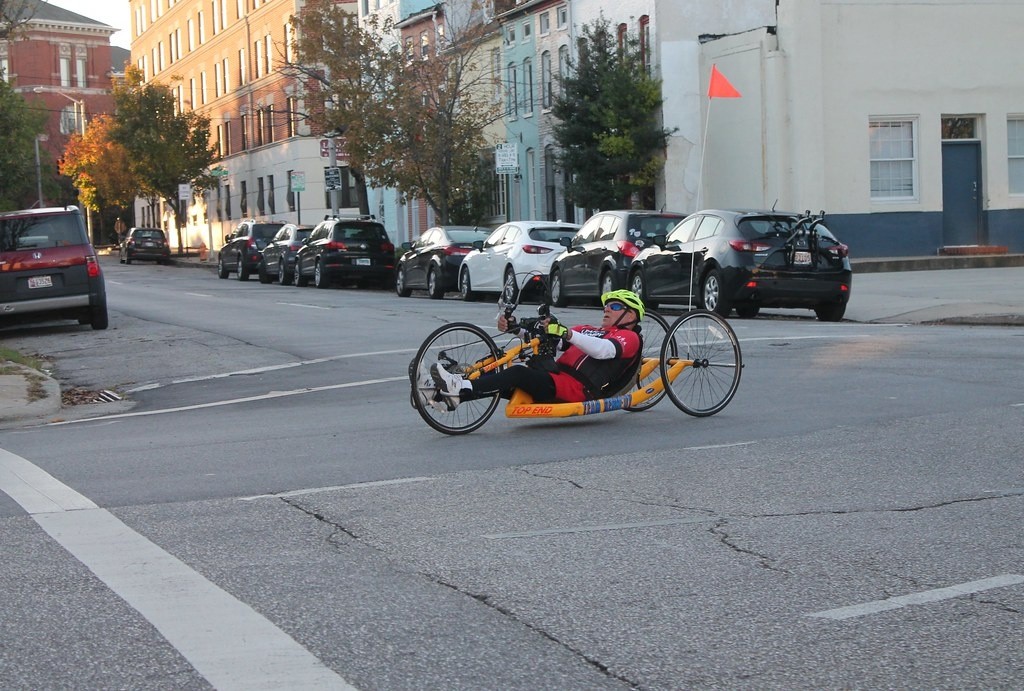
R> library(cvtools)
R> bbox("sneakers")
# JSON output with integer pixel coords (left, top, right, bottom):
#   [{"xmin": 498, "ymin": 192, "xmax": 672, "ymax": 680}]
[
  {"xmin": 438, "ymin": 351, "xmax": 470, "ymax": 379},
  {"xmin": 430, "ymin": 362, "xmax": 463, "ymax": 410}
]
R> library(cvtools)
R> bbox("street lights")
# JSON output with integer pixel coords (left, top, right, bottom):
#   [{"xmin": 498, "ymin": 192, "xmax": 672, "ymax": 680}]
[{"xmin": 34, "ymin": 87, "xmax": 93, "ymax": 245}]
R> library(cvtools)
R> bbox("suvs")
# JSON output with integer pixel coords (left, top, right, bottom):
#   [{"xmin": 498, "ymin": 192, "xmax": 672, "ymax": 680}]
[
  {"xmin": 217, "ymin": 219, "xmax": 291, "ymax": 282},
  {"xmin": 293, "ymin": 213, "xmax": 396, "ymax": 289}
]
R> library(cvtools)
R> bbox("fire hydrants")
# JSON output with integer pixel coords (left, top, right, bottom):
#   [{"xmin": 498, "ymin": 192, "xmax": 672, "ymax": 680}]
[{"xmin": 198, "ymin": 242, "xmax": 208, "ymax": 261}]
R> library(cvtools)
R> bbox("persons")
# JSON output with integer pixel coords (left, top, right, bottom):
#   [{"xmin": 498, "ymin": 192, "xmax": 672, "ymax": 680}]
[{"xmin": 408, "ymin": 289, "xmax": 646, "ymax": 410}]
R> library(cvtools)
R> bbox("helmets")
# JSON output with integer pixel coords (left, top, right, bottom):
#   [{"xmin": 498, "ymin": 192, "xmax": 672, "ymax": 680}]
[{"xmin": 601, "ymin": 289, "xmax": 645, "ymax": 322}]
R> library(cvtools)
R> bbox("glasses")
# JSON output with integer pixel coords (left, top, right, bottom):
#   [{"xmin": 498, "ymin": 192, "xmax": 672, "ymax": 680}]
[{"xmin": 604, "ymin": 303, "xmax": 633, "ymax": 314}]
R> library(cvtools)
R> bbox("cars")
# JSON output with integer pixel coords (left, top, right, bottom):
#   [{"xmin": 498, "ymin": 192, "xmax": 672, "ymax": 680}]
[
  {"xmin": 0, "ymin": 205, "xmax": 109, "ymax": 330},
  {"xmin": 626, "ymin": 209, "xmax": 854, "ymax": 321},
  {"xmin": 546, "ymin": 210, "xmax": 690, "ymax": 308},
  {"xmin": 394, "ymin": 226, "xmax": 495, "ymax": 299},
  {"xmin": 457, "ymin": 219, "xmax": 584, "ymax": 305},
  {"xmin": 119, "ymin": 227, "xmax": 170, "ymax": 266},
  {"xmin": 258, "ymin": 223, "xmax": 317, "ymax": 286}
]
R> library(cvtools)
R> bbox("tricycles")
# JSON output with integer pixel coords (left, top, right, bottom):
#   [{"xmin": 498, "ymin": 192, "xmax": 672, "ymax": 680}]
[{"xmin": 411, "ymin": 269, "xmax": 745, "ymax": 436}]
